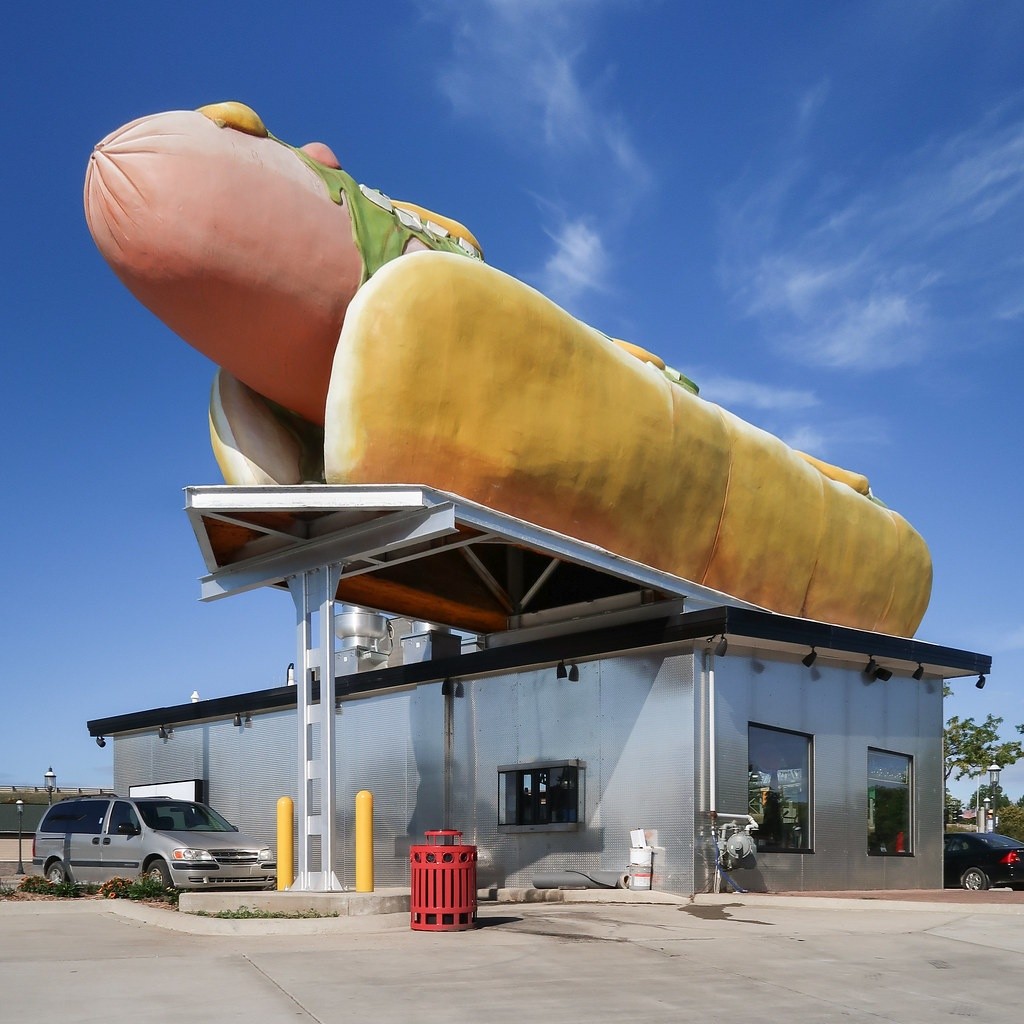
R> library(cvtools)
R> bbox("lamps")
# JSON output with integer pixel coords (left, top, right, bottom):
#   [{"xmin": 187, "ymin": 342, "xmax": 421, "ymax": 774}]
[
  {"xmin": 557, "ymin": 659, "xmax": 568, "ymax": 678},
  {"xmin": 865, "ymin": 653, "xmax": 893, "ymax": 681},
  {"xmin": 912, "ymin": 662, "xmax": 924, "ymax": 680},
  {"xmin": 44, "ymin": 766, "xmax": 56, "ymax": 793},
  {"xmin": 975, "ymin": 673, "xmax": 986, "ymax": 689},
  {"xmin": 16, "ymin": 798, "xmax": 24, "ymax": 815},
  {"xmin": 809, "ymin": 666, "xmax": 822, "ymax": 683},
  {"xmin": 159, "ymin": 723, "xmax": 166, "ymax": 739},
  {"xmin": 334, "ymin": 698, "xmax": 342, "ymax": 715},
  {"xmin": 556, "ymin": 767, "xmax": 576, "ymax": 793},
  {"xmin": 801, "ymin": 645, "xmax": 817, "ymax": 668},
  {"xmin": 233, "ymin": 711, "xmax": 242, "ymax": 726},
  {"xmin": 748, "ymin": 656, "xmax": 766, "ymax": 674},
  {"xmin": 714, "ymin": 632, "xmax": 728, "ymax": 658},
  {"xmin": 441, "ymin": 678, "xmax": 454, "ymax": 696},
  {"xmin": 95, "ymin": 736, "xmax": 106, "ymax": 748}
]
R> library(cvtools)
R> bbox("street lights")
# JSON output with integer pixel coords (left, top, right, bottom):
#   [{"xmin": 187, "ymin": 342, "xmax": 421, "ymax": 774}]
[
  {"xmin": 16, "ymin": 799, "xmax": 26, "ymax": 874},
  {"xmin": 987, "ymin": 759, "xmax": 1002, "ymax": 833},
  {"xmin": 44, "ymin": 766, "xmax": 57, "ymax": 806},
  {"xmin": 983, "ymin": 796, "xmax": 991, "ymax": 833}
]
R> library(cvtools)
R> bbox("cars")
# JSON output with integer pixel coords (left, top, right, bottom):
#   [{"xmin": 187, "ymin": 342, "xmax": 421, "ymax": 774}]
[{"xmin": 943, "ymin": 833, "xmax": 1024, "ymax": 892}]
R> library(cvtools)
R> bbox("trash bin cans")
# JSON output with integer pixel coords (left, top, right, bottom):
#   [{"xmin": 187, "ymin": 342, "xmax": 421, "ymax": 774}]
[{"xmin": 410, "ymin": 829, "xmax": 478, "ymax": 932}]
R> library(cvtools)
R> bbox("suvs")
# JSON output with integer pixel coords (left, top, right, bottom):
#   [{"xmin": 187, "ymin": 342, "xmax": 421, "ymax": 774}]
[{"xmin": 32, "ymin": 793, "xmax": 277, "ymax": 896}]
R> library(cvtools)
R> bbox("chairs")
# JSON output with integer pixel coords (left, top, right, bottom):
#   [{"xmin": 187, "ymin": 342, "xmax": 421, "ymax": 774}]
[{"xmin": 155, "ymin": 817, "xmax": 175, "ymax": 831}]
